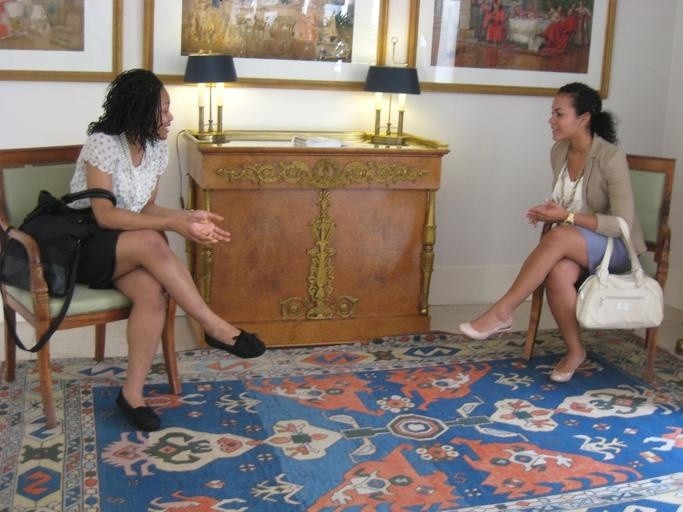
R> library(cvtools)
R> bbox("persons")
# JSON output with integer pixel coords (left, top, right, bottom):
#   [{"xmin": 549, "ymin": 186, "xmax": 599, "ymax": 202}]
[
  {"xmin": 65, "ymin": 68, "xmax": 265, "ymax": 431},
  {"xmin": 470, "ymin": 0, "xmax": 592, "ymax": 56},
  {"xmin": 457, "ymin": 83, "xmax": 647, "ymax": 383}
]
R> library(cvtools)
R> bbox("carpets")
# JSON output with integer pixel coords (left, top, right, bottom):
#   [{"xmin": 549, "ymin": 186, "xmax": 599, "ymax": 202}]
[{"xmin": 0, "ymin": 329, "xmax": 682, "ymax": 512}]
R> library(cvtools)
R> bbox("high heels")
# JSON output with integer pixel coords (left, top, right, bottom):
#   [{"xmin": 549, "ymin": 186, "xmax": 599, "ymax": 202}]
[
  {"xmin": 548, "ymin": 347, "xmax": 589, "ymax": 384},
  {"xmin": 459, "ymin": 321, "xmax": 513, "ymax": 342}
]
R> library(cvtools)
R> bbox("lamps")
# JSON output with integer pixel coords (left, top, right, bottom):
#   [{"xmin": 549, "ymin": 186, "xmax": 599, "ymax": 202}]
[
  {"xmin": 185, "ymin": 29, "xmax": 238, "ymax": 145},
  {"xmin": 363, "ymin": 36, "xmax": 420, "ymax": 146}
]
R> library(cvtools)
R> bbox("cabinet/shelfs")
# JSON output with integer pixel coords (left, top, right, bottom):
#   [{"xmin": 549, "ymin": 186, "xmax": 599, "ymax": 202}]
[{"xmin": 183, "ymin": 130, "xmax": 450, "ymax": 347}]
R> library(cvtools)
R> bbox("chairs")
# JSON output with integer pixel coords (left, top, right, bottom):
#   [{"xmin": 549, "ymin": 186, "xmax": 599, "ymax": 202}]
[
  {"xmin": 522, "ymin": 153, "xmax": 675, "ymax": 381},
  {"xmin": 0, "ymin": 145, "xmax": 182, "ymax": 425}
]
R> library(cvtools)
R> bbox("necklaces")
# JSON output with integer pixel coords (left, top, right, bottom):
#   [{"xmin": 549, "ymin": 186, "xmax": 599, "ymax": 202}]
[
  {"xmin": 120, "ymin": 132, "xmax": 147, "ymax": 174},
  {"xmin": 561, "ymin": 133, "xmax": 595, "ymax": 210}
]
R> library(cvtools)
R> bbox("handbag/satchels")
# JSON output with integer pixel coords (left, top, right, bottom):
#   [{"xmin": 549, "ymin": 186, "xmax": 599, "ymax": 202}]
[
  {"xmin": 576, "ymin": 217, "xmax": 666, "ymax": 331},
  {"xmin": 0, "ymin": 188, "xmax": 117, "ymax": 353}
]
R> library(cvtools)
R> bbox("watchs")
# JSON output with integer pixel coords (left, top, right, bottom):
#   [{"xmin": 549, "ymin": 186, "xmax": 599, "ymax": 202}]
[{"xmin": 564, "ymin": 212, "xmax": 576, "ymax": 226}]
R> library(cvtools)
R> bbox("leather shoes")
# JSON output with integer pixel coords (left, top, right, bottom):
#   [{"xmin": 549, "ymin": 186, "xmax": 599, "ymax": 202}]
[
  {"xmin": 205, "ymin": 329, "xmax": 267, "ymax": 359},
  {"xmin": 115, "ymin": 386, "xmax": 162, "ymax": 432}
]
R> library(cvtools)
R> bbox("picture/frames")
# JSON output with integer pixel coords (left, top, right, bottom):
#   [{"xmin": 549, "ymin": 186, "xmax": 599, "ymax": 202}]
[
  {"xmin": 143, "ymin": 0, "xmax": 389, "ymax": 93},
  {"xmin": 0, "ymin": 0, "xmax": 125, "ymax": 83},
  {"xmin": 407, "ymin": 0, "xmax": 619, "ymax": 98}
]
